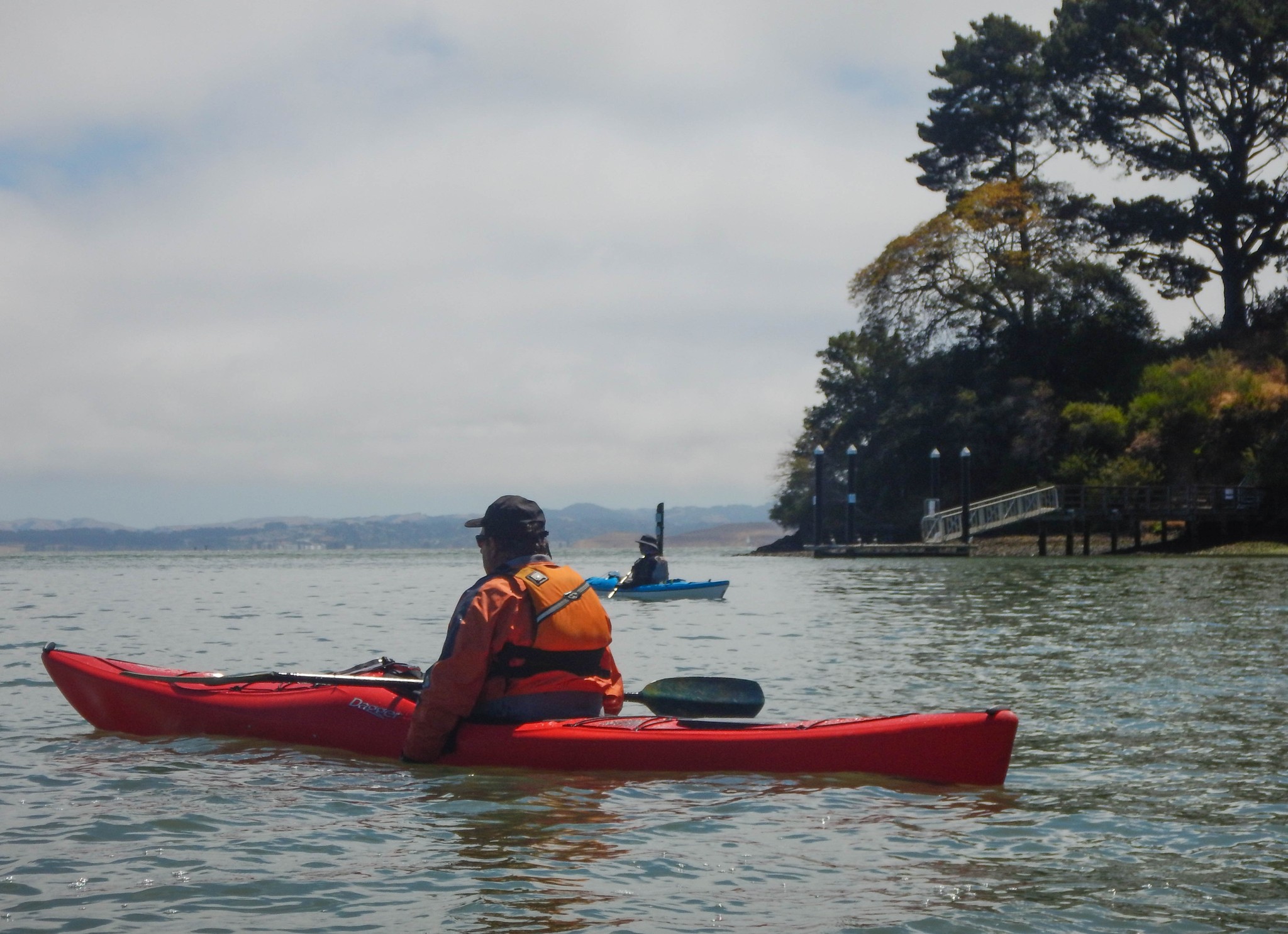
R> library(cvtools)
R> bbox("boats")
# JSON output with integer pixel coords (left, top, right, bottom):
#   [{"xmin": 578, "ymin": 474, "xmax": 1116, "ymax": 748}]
[
  {"xmin": 584, "ymin": 571, "xmax": 731, "ymax": 600},
  {"xmin": 37, "ymin": 636, "xmax": 1023, "ymax": 788}
]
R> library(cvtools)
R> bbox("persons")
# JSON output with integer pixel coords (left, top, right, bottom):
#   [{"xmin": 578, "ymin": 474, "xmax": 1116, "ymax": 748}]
[
  {"xmin": 614, "ymin": 534, "xmax": 658, "ymax": 589},
  {"xmin": 400, "ymin": 495, "xmax": 624, "ymax": 765}
]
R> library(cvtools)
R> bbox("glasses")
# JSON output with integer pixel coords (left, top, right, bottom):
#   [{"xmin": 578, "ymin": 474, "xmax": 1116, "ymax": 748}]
[{"xmin": 475, "ymin": 532, "xmax": 491, "ymax": 549}]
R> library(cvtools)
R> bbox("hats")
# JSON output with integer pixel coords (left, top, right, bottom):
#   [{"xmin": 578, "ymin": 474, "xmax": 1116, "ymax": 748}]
[
  {"xmin": 635, "ymin": 534, "xmax": 659, "ymax": 551},
  {"xmin": 464, "ymin": 494, "xmax": 549, "ymax": 537}
]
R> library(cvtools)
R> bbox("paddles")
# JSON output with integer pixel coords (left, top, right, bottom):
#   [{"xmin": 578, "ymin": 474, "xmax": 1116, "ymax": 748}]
[
  {"xmin": 117, "ymin": 668, "xmax": 765, "ymax": 719},
  {"xmin": 607, "ymin": 555, "xmax": 645, "ymax": 599}
]
